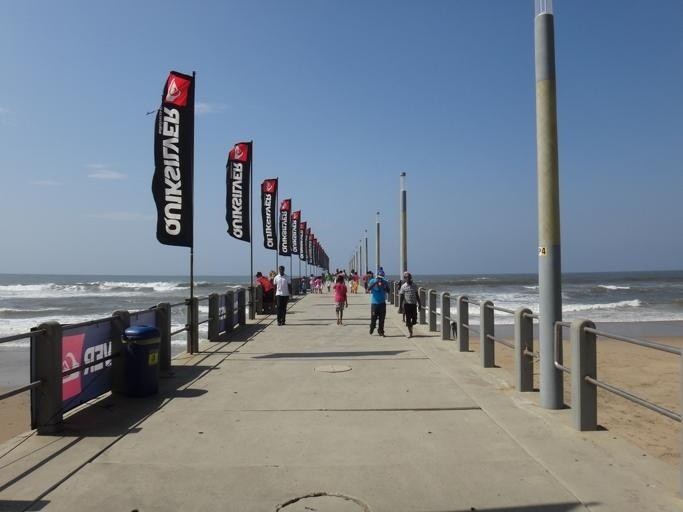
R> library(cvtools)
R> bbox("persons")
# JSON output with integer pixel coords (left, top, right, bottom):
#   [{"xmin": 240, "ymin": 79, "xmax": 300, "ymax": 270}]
[
  {"xmin": 253, "ymin": 271, "xmax": 274, "ymax": 315},
  {"xmin": 397, "ymin": 271, "xmax": 407, "ymax": 314},
  {"xmin": 366, "ymin": 266, "xmax": 390, "ymax": 337},
  {"xmin": 298, "ymin": 267, "xmax": 374, "ymax": 295},
  {"xmin": 331, "ymin": 275, "xmax": 348, "ymax": 326},
  {"xmin": 396, "ymin": 271, "xmax": 421, "ymax": 339},
  {"xmin": 267, "ymin": 270, "xmax": 277, "ymax": 314},
  {"xmin": 271, "ymin": 265, "xmax": 293, "ymax": 326}
]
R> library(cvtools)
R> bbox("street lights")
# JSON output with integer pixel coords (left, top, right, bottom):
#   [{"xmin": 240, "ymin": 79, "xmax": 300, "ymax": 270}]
[
  {"xmin": 347, "ymin": 229, "xmax": 369, "ymax": 277},
  {"xmin": 530, "ymin": 0, "xmax": 570, "ymax": 413},
  {"xmin": 396, "ymin": 171, "xmax": 410, "ymax": 314},
  {"xmin": 375, "ymin": 210, "xmax": 382, "ymax": 279}
]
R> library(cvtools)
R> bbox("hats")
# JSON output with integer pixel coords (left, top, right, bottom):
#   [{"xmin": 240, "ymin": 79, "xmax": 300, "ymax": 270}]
[
  {"xmin": 256, "ymin": 272, "xmax": 261, "ymax": 276},
  {"xmin": 377, "ymin": 271, "xmax": 385, "ymax": 279}
]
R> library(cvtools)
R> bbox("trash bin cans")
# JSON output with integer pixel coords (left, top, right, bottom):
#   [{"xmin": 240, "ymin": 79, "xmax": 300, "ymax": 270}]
[{"xmin": 119, "ymin": 324, "xmax": 160, "ymax": 398}]
